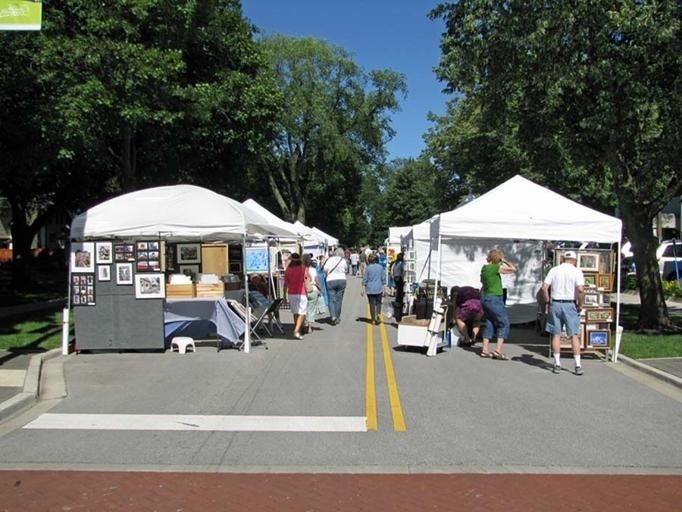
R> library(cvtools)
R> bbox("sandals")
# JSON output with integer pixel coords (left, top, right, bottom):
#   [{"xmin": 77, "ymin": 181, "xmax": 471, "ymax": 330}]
[
  {"xmin": 481, "ymin": 352, "xmax": 494, "ymax": 358},
  {"xmin": 492, "ymin": 350, "xmax": 509, "ymax": 360},
  {"xmin": 293, "ymin": 332, "xmax": 304, "ymax": 340}
]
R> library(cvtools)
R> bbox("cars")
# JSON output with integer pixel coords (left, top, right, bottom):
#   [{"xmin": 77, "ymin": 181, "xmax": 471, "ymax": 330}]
[{"xmin": 554, "ymin": 237, "xmax": 682, "ymax": 281}]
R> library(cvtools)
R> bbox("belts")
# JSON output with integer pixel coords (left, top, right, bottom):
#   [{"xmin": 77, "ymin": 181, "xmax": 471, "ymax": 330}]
[{"xmin": 552, "ymin": 300, "xmax": 572, "ymax": 302}]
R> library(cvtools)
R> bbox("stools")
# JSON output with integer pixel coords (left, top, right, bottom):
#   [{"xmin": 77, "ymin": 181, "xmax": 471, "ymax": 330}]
[{"xmin": 170, "ymin": 336, "xmax": 196, "ymax": 354}]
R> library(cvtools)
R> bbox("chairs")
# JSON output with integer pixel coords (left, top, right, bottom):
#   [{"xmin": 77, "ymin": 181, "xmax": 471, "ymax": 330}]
[{"xmin": 231, "ymin": 290, "xmax": 285, "ymax": 351}]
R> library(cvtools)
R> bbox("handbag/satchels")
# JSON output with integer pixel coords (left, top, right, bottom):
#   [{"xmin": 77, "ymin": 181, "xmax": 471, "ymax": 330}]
[{"xmin": 304, "ymin": 274, "xmax": 313, "ymax": 293}]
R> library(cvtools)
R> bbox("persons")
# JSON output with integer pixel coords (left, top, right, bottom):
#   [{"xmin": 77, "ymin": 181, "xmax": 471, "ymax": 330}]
[
  {"xmin": 447, "ymin": 285, "xmax": 484, "ymax": 348},
  {"xmin": 478, "ymin": 249, "xmax": 517, "ymax": 360},
  {"xmin": 249, "ymin": 273, "xmax": 283, "ymax": 329},
  {"xmin": 541, "ymin": 251, "xmax": 587, "ymax": 375},
  {"xmin": 283, "ymin": 246, "xmax": 406, "ymax": 339}
]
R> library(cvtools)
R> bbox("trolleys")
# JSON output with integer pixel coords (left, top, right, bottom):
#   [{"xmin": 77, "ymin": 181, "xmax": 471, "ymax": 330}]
[{"xmin": 397, "ymin": 304, "xmax": 449, "ymax": 353}]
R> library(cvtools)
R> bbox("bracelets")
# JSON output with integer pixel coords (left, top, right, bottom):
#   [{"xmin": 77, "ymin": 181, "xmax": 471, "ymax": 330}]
[
  {"xmin": 579, "ymin": 302, "xmax": 584, "ymax": 307},
  {"xmin": 544, "ymin": 300, "xmax": 550, "ymax": 304}
]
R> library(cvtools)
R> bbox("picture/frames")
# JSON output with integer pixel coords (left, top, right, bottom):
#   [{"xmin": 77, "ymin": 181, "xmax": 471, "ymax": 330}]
[
  {"xmin": 242, "ymin": 243, "xmax": 299, "ymax": 277},
  {"xmin": 556, "ymin": 253, "xmax": 614, "ymax": 348},
  {"xmin": 69, "ymin": 239, "xmax": 202, "ymax": 306}
]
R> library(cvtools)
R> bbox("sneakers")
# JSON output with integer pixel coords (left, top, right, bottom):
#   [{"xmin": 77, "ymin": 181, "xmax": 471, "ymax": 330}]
[
  {"xmin": 575, "ymin": 366, "xmax": 582, "ymax": 375},
  {"xmin": 553, "ymin": 364, "xmax": 561, "ymax": 374}
]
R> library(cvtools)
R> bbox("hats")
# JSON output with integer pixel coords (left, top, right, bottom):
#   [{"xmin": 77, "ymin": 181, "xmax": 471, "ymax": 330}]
[
  {"xmin": 564, "ymin": 251, "xmax": 577, "ymax": 260},
  {"xmin": 288, "ymin": 255, "xmax": 300, "ymax": 261}
]
